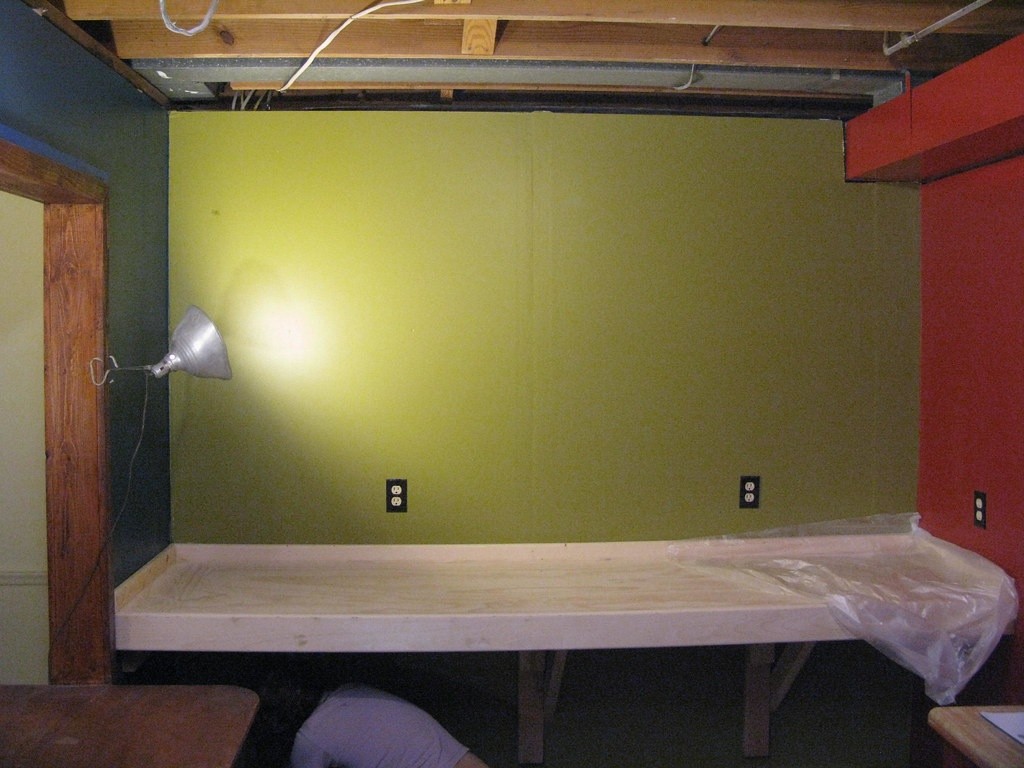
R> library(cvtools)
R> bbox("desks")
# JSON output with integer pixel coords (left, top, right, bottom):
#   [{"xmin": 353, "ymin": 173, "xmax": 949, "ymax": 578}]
[
  {"xmin": 926, "ymin": 705, "xmax": 1023, "ymax": 768},
  {"xmin": 0, "ymin": 684, "xmax": 261, "ymax": 768},
  {"xmin": 112, "ymin": 554, "xmax": 1016, "ymax": 764}
]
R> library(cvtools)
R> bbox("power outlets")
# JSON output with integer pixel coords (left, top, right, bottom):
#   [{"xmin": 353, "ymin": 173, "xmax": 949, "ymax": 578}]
[
  {"xmin": 386, "ymin": 478, "xmax": 408, "ymax": 514},
  {"xmin": 738, "ymin": 475, "xmax": 761, "ymax": 509},
  {"xmin": 972, "ymin": 490, "xmax": 988, "ymax": 531}
]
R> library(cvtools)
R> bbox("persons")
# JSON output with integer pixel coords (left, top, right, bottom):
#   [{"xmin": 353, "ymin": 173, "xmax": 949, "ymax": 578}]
[{"xmin": 272, "ymin": 666, "xmax": 492, "ymax": 768}]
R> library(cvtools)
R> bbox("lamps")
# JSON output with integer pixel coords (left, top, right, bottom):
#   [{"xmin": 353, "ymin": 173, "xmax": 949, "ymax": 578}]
[{"xmin": 90, "ymin": 301, "xmax": 234, "ymax": 385}]
